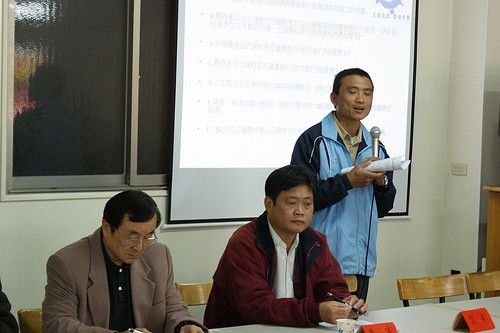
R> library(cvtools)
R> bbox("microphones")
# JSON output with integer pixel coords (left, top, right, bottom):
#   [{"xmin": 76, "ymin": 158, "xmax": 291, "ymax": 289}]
[{"xmin": 370, "ymin": 126, "xmax": 381, "ymax": 157}]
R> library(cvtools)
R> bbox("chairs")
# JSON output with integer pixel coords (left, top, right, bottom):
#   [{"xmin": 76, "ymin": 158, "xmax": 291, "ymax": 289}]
[
  {"xmin": 397, "ymin": 274, "xmax": 466, "ymax": 307},
  {"xmin": 17, "ymin": 308, "xmax": 44, "ymax": 333},
  {"xmin": 175, "ymin": 282, "xmax": 212, "ymax": 306},
  {"xmin": 465, "ymin": 271, "xmax": 500, "ymax": 300},
  {"xmin": 343, "ymin": 274, "xmax": 357, "ymax": 292}
]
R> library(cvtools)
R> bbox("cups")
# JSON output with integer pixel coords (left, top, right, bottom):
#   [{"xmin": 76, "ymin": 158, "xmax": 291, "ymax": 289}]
[{"xmin": 336, "ymin": 318, "xmax": 356, "ymax": 333}]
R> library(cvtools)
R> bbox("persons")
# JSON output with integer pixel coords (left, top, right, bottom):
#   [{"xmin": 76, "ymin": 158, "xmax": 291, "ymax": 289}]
[
  {"xmin": 287, "ymin": 68, "xmax": 397, "ymax": 303},
  {"xmin": 42, "ymin": 190, "xmax": 209, "ymax": 333},
  {"xmin": 0, "ymin": 278, "xmax": 20, "ymax": 333},
  {"xmin": 203, "ymin": 165, "xmax": 368, "ymax": 329}
]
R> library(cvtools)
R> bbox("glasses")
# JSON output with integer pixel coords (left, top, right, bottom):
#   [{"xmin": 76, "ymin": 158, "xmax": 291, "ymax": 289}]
[{"xmin": 113, "ymin": 227, "xmax": 159, "ymax": 247}]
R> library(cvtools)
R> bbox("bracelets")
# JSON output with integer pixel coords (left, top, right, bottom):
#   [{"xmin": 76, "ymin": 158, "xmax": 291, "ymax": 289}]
[{"xmin": 384, "ymin": 175, "xmax": 388, "ymax": 188}]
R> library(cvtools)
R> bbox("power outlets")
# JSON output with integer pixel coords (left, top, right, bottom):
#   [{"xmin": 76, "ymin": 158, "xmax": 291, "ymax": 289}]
[{"xmin": 451, "ymin": 163, "xmax": 467, "ymax": 176}]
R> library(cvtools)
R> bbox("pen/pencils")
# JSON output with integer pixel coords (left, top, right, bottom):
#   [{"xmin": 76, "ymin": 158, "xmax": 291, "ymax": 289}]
[
  {"xmin": 128, "ymin": 329, "xmax": 142, "ymax": 333},
  {"xmin": 328, "ymin": 292, "xmax": 362, "ymax": 317}
]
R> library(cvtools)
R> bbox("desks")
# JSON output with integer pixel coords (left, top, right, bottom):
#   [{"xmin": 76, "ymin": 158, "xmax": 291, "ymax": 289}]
[{"xmin": 209, "ymin": 296, "xmax": 500, "ymax": 333}]
